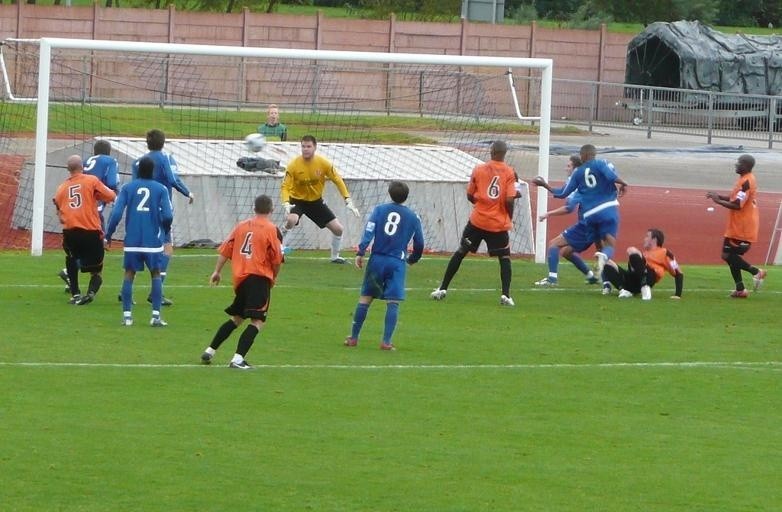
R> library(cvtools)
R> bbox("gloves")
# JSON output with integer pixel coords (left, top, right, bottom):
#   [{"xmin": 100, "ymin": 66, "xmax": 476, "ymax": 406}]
[
  {"xmin": 344, "ymin": 197, "xmax": 360, "ymax": 218},
  {"xmin": 281, "ymin": 202, "xmax": 296, "ymax": 222}
]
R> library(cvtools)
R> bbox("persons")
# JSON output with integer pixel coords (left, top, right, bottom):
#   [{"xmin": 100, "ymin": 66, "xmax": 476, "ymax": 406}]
[
  {"xmin": 428, "ymin": 141, "xmax": 521, "ymax": 306},
  {"xmin": 705, "ymin": 153, "xmax": 767, "ymax": 298},
  {"xmin": 532, "ymin": 143, "xmax": 628, "ymax": 296},
  {"xmin": 343, "ymin": 180, "xmax": 425, "ymax": 351},
  {"xmin": 256, "ymin": 103, "xmax": 288, "ymax": 142},
  {"xmin": 605, "ymin": 228, "xmax": 683, "ymax": 301},
  {"xmin": 535, "ymin": 155, "xmax": 599, "ymax": 287},
  {"xmin": 52, "ymin": 129, "xmax": 194, "ymax": 328},
  {"xmin": 196, "ymin": 193, "xmax": 286, "ymax": 371},
  {"xmin": 279, "ymin": 134, "xmax": 360, "ymax": 265}
]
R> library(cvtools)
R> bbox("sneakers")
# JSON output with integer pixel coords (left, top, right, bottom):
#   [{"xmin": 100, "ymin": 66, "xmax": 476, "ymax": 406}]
[
  {"xmin": 229, "ymin": 359, "xmax": 252, "ymax": 370},
  {"xmin": 118, "ymin": 292, "xmax": 136, "ymax": 305},
  {"xmin": 150, "ymin": 316, "xmax": 168, "ymax": 328},
  {"xmin": 729, "ymin": 289, "xmax": 748, "ymax": 298},
  {"xmin": 147, "ymin": 295, "xmax": 172, "ymax": 306},
  {"xmin": 201, "ymin": 351, "xmax": 213, "ymax": 365},
  {"xmin": 57, "ymin": 268, "xmax": 93, "ymax": 306},
  {"xmin": 120, "ymin": 318, "xmax": 133, "ymax": 326},
  {"xmin": 344, "ymin": 337, "xmax": 357, "ymax": 346},
  {"xmin": 431, "ymin": 288, "xmax": 446, "ymax": 299},
  {"xmin": 583, "ymin": 250, "xmax": 605, "ymax": 287},
  {"xmin": 752, "ymin": 268, "xmax": 768, "ymax": 292},
  {"xmin": 618, "ymin": 289, "xmax": 633, "ymax": 298},
  {"xmin": 641, "ymin": 285, "xmax": 652, "ymax": 300},
  {"xmin": 501, "ymin": 294, "xmax": 514, "ymax": 306},
  {"xmin": 602, "ymin": 288, "xmax": 611, "ymax": 295},
  {"xmin": 380, "ymin": 342, "xmax": 396, "ymax": 350},
  {"xmin": 331, "ymin": 257, "xmax": 345, "ymax": 264},
  {"xmin": 534, "ymin": 277, "xmax": 558, "ymax": 286}
]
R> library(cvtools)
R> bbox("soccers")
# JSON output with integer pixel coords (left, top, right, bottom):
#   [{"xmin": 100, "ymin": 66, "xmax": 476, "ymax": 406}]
[{"xmin": 246, "ymin": 133, "xmax": 266, "ymax": 152}]
